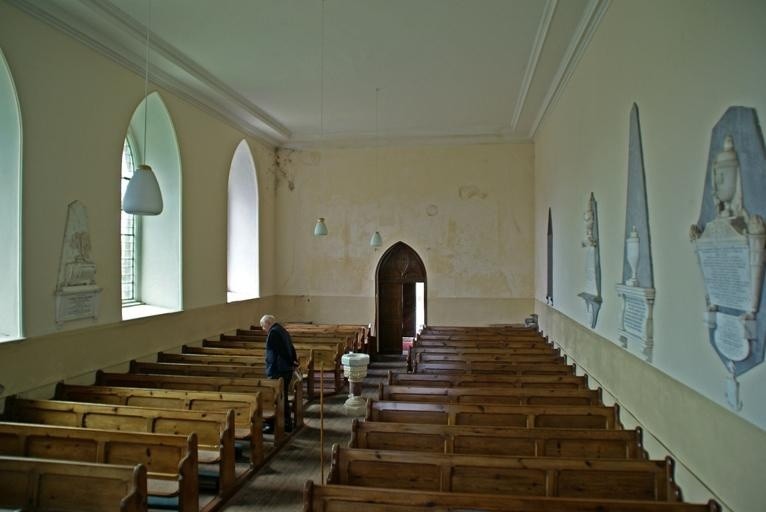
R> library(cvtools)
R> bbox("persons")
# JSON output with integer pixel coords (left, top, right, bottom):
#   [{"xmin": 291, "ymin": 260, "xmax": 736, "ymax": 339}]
[{"xmin": 259, "ymin": 313, "xmax": 300, "ymax": 434}]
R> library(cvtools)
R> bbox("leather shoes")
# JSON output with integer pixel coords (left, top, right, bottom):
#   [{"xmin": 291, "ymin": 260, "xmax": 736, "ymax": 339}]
[{"xmin": 262, "ymin": 426, "xmax": 273, "ymax": 434}]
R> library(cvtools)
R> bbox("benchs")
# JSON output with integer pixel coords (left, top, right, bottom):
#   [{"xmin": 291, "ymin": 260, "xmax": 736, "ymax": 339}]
[{"xmin": 0, "ymin": 321, "xmax": 722, "ymax": 510}]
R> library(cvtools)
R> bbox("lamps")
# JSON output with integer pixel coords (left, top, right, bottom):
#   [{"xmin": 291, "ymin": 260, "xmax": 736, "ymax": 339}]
[
  {"xmin": 313, "ymin": 1, "xmax": 328, "ymax": 237},
  {"xmin": 120, "ymin": 0, "xmax": 165, "ymax": 216},
  {"xmin": 369, "ymin": 88, "xmax": 382, "ymax": 247}
]
